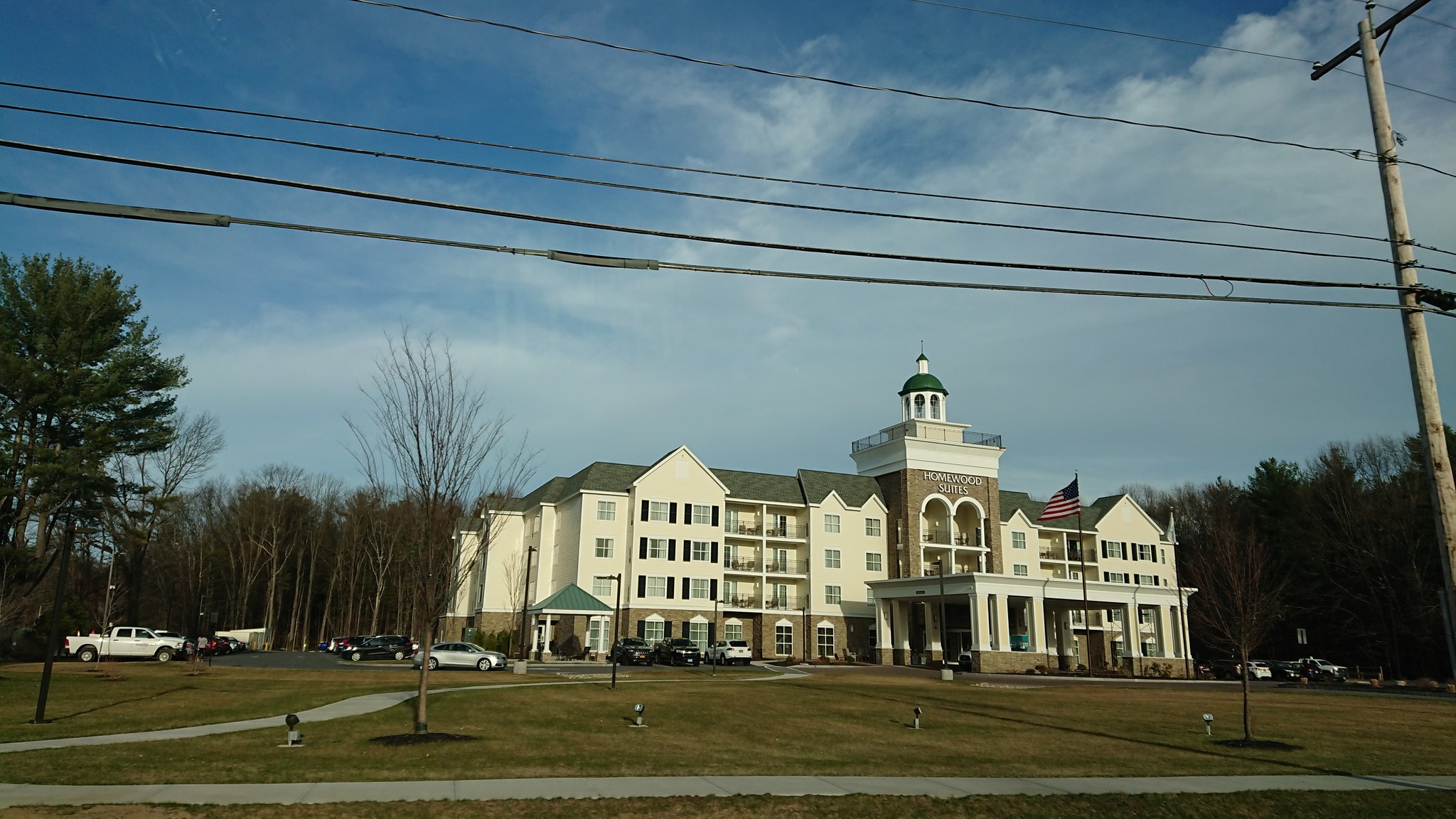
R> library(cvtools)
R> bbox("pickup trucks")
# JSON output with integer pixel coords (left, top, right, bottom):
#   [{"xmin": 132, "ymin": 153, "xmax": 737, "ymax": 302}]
[
  {"xmin": 64, "ymin": 627, "xmax": 188, "ymax": 663},
  {"xmin": 653, "ymin": 637, "xmax": 701, "ymax": 667},
  {"xmin": 1282, "ymin": 658, "xmax": 1349, "ymax": 683}
]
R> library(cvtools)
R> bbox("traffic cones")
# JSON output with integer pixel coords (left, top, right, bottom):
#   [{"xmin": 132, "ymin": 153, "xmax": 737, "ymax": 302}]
[
  {"xmin": 29, "ymin": 525, "xmax": 99, "ymax": 724},
  {"xmin": 77, "ymin": 631, "xmax": 80, "ymax": 637}
]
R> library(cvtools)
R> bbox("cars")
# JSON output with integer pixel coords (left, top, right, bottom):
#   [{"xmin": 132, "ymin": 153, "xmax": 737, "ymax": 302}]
[{"xmin": 412, "ymin": 642, "xmax": 508, "ymax": 671}]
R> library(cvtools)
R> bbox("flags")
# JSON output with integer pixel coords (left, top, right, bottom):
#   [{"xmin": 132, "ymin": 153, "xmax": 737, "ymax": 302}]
[
  {"xmin": 1166, "ymin": 510, "xmax": 1176, "ymax": 544},
  {"xmin": 1036, "ymin": 479, "xmax": 1080, "ymax": 521}
]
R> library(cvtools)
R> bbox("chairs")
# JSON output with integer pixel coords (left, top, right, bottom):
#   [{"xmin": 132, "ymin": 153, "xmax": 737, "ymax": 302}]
[
  {"xmin": 842, "ymin": 648, "xmax": 869, "ymax": 662},
  {"xmin": 739, "ymin": 525, "xmax": 762, "ymax": 535},
  {"xmin": 732, "ymin": 596, "xmax": 754, "ymax": 607},
  {"xmin": 926, "ymin": 534, "xmax": 934, "ymax": 542},
  {"xmin": 771, "ymin": 598, "xmax": 779, "ymax": 610},
  {"xmin": 772, "ymin": 563, "xmax": 779, "ymax": 572},
  {"xmin": 732, "ymin": 560, "xmax": 755, "ymax": 570},
  {"xmin": 556, "ymin": 646, "xmax": 591, "ymax": 661},
  {"xmin": 924, "ymin": 569, "xmax": 929, "ymax": 576},
  {"xmin": 766, "ymin": 527, "xmax": 783, "ymax": 537}
]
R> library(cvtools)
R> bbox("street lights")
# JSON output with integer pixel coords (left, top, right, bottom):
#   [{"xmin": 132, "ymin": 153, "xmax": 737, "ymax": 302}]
[
  {"xmin": 517, "ymin": 546, "xmax": 537, "ymax": 662},
  {"xmin": 197, "ymin": 594, "xmax": 210, "ymax": 635},
  {"xmin": 713, "ymin": 598, "xmax": 731, "ymax": 676},
  {"xmin": 792, "ymin": 607, "xmax": 806, "ymax": 664},
  {"xmin": 102, "ymin": 550, "xmax": 128, "ymax": 633},
  {"xmin": 931, "ymin": 559, "xmax": 951, "ymax": 670},
  {"xmin": 596, "ymin": 574, "xmax": 622, "ymax": 688}
]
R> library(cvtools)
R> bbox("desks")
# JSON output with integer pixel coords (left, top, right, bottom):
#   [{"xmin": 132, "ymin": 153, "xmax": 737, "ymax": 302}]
[
  {"xmin": 741, "ymin": 599, "xmax": 747, "ymax": 608},
  {"xmin": 925, "ymin": 571, "xmax": 933, "ymax": 576},
  {"xmin": 767, "ymin": 529, "xmax": 774, "ymax": 536},
  {"xmin": 740, "ymin": 563, "xmax": 748, "ymax": 571},
  {"xmin": 766, "ymin": 565, "xmax": 772, "ymax": 572},
  {"xmin": 923, "ymin": 535, "xmax": 928, "ymax": 542}
]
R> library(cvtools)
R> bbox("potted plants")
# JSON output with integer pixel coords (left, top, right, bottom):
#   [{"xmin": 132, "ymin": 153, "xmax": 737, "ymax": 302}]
[{"xmin": 918, "ymin": 654, "xmax": 927, "ymax": 665}]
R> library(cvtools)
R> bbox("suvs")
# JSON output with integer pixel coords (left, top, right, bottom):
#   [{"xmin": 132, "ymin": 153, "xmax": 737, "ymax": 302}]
[
  {"xmin": 704, "ymin": 639, "xmax": 753, "ymax": 666},
  {"xmin": 139, "ymin": 630, "xmax": 246, "ymax": 659},
  {"xmin": 1193, "ymin": 659, "xmax": 1322, "ymax": 683},
  {"xmin": 412, "ymin": 642, "xmax": 420, "ymax": 650},
  {"xmin": 610, "ymin": 636, "xmax": 654, "ymax": 666},
  {"xmin": 340, "ymin": 635, "xmax": 414, "ymax": 661},
  {"xmin": 317, "ymin": 635, "xmax": 377, "ymax": 655}
]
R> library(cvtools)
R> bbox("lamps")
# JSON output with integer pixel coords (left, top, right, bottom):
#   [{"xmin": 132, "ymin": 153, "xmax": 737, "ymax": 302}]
[
  {"xmin": 850, "ymin": 625, "xmax": 853, "ymax": 633},
  {"xmin": 934, "ymin": 614, "xmax": 937, "ymax": 622},
  {"xmin": 884, "ymin": 611, "xmax": 888, "ymax": 619}
]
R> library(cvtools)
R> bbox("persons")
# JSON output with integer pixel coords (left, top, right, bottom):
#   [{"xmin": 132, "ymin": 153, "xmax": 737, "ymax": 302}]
[
  {"xmin": 1078, "ymin": 550, "xmax": 1081, "ymax": 561},
  {"xmin": 194, "ymin": 635, "xmax": 207, "ymax": 662}
]
